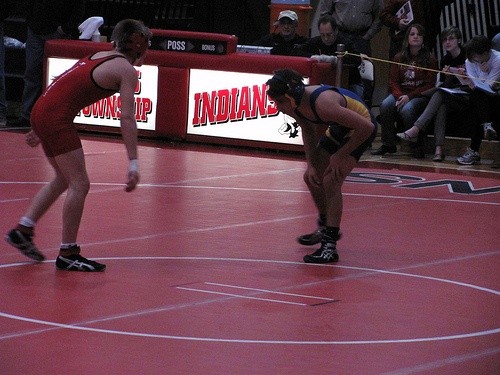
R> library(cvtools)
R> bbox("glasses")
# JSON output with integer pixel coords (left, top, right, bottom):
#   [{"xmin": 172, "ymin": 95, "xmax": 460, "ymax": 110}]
[{"xmin": 442, "ymin": 36, "xmax": 455, "ymax": 43}]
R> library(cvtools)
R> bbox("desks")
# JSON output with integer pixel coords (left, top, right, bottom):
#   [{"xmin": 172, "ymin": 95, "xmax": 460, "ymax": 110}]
[{"xmin": 43, "ymin": 39, "xmax": 337, "ymax": 153}]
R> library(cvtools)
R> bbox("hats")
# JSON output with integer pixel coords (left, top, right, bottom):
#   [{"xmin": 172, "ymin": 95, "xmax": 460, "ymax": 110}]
[{"xmin": 278, "ymin": 10, "xmax": 298, "ymax": 21}]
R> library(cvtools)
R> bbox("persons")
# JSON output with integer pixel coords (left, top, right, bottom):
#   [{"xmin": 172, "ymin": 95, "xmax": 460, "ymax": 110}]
[
  {"xmin": 377, "ymin": 0, "xmax": 456, "ymax": 65},
  {"xmin": 369, "ymin": 23, "xmax": 500, "ymax": 165},
  {"xmin": 253, "ymin": 0, "xmax": 385, "ymax": 57},
  {"xmin": 3, "ymin": 18, "xmax": 153, "ymax": 272},
  {"xmin": 265, "ymin": 68, "xmax": 378, "ymax": 264},
  {"xmin": 6, "ymin": 0, "xmax": 87, "ymax": 127}
]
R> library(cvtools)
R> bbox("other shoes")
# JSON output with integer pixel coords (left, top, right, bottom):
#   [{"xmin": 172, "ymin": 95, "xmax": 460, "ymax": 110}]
[
  {"xmin": 415, "ymin": 147, "xmax": 424, "ymax": 158},
  {"xmin": 432, "ymin": 152, "xmax": 445, "ymax": 161},
  {"xmin": 397, "ymin": 132, "xmax": 417, "ymax": 144},
  {"xmin": 370, "ymin": 145, "xmax": 398, "ymax": 155},
  {"xmin": 6, "ymin": 116, "xmax": 31, "ymax": 127}
]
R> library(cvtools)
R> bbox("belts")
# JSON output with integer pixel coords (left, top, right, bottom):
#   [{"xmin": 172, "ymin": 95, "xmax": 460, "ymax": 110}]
[{"xmin": 339, "ymin": 27, "xmax": 360, "ymax": 36}]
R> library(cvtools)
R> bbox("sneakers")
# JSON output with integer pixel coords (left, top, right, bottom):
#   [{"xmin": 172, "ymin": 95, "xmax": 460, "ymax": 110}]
[
  {"xmin": 457, "ymin": 150, "xmax": 480, "ymax": 164},
  {"xmin": 484, "ymin": 123, "xmax": 495, "ymax": 141},
  {"xmin": 303, "ymin": 235, "xmax": 339, "ymax": 263},
  {"xmin": 56, "ymin": 253, "xmax": 105, "ymax": 272},
  {"xmin": 299, "ymin": 221, "xmax": 340, "ymax": 245},
  {"xmin": 5, "ymin": 228, "xmax": 45, "ymax": 261}
]
